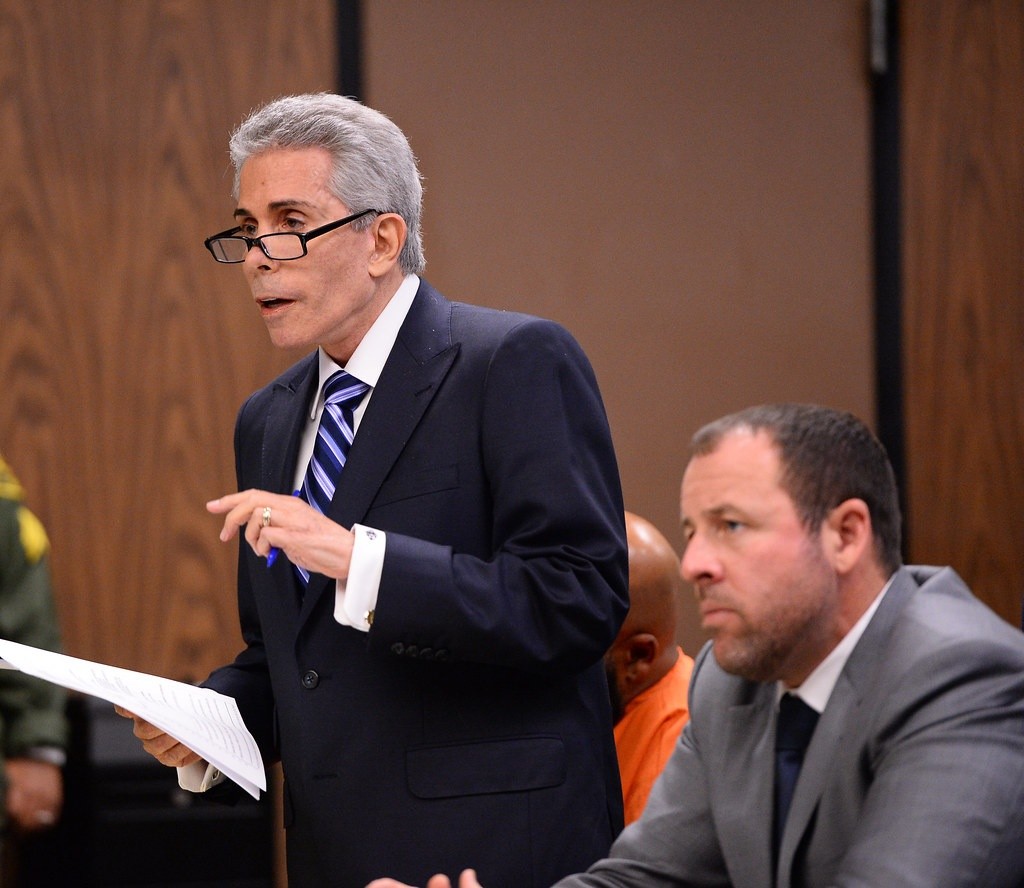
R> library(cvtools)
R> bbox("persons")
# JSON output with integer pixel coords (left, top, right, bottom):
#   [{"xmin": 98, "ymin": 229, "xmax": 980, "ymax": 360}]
[
  {"xmin": 370, "ymin": 403, "xmax": 1024, "ymax": 888},
  {"xmin": 114, "ymin": 93, "xmax": 631, "ymax": 888},
  {"xmin": 604, "ymin": 510, "xmax": 695, "ymax": 828},
  {"xmin": 0, "ymin": 454, "xmax": 65, "ymax": 888}
]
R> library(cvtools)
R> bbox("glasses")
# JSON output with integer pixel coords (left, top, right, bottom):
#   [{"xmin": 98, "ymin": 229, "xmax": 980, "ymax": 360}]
[{"xmin": 201, "ymin": 210, "xmax": 376, "ymax": 265}]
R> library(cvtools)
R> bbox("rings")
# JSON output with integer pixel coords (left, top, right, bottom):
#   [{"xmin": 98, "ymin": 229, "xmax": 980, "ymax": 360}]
[
  {"xmin": 34, "ymin": 809, "xmax": 56, "ymax": 823},
  {"xmin": 262, "ymin": 506, "xmax": 271, "ymax": 527}
]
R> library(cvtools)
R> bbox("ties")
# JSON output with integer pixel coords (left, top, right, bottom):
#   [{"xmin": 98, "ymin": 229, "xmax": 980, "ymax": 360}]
[
  {"xmin": 767, "ymin": 693, "xmax": 821, "ymax": 857},
  {"xmin": 293, "ymin": 370, "xmax": 371, "ymax": 615}
]
